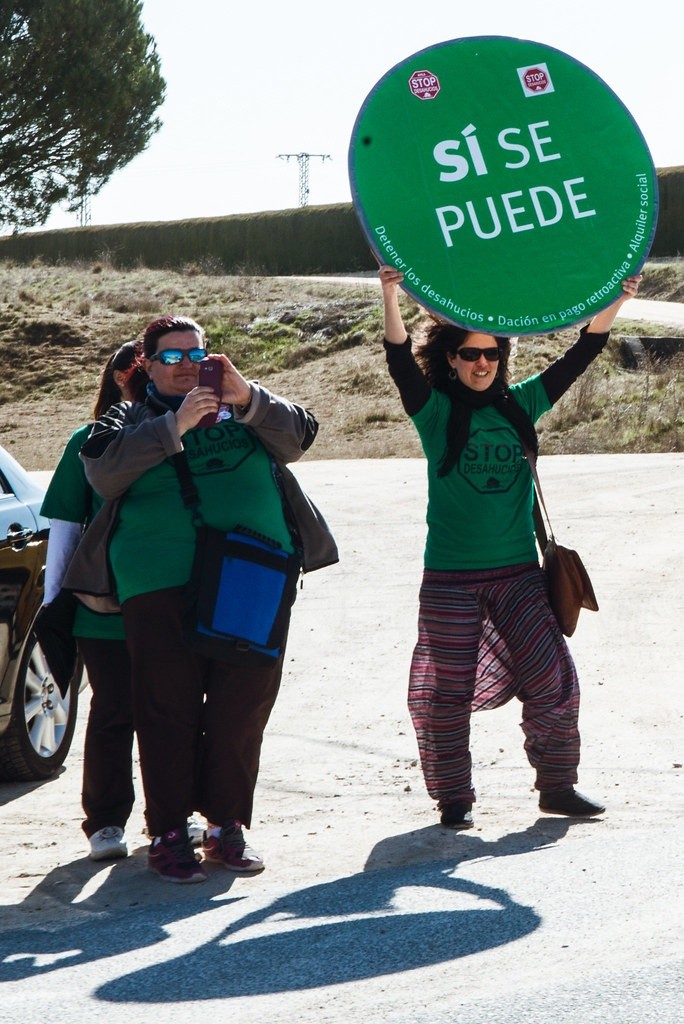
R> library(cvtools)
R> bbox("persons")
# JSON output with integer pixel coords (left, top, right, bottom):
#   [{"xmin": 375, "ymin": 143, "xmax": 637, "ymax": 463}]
[
  {"xmin": 39, "ymin": 316, "xmax": 340, "ymax": 883},
  {"xmin": 378, "ymin": 265, "xmax": 643, "ymax": 829}
]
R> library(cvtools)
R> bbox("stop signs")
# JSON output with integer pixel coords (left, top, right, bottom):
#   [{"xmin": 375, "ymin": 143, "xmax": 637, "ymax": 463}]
[
  {"xmin": 517, "ymin": 62, "xmax": 555, "ymax": 98},
  {"xmin": 407, "ymin": 69, "xmax": 441, "ymax": 100}
]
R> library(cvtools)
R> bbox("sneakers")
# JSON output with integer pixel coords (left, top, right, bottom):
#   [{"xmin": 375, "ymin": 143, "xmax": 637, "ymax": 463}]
[
  {"xmin": 441, "ymin": 805, "xmax": 474, "ymax": 829},
  {"xmin": 147, "ymin": 826, "xmax": 207, "ymax": 882},
  {"xmin": 540, "ymin": 788, "xmax": 606, "ymax": 817},
  {"xmin": 89, "ymin": 826, "xmax": 128, "ymax": 860},
  {"xmin": 186, "ymin": 817, "xmax": 207, "ymax": 845},
  {"xmin": 202, "ymin": 824, "xmax": 264, "ymax": 871}
]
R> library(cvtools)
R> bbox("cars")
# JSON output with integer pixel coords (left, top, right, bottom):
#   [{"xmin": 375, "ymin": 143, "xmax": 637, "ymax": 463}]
[{"xmin": 0, "ymin": 443, "xmax": 89, "ymax": 785}]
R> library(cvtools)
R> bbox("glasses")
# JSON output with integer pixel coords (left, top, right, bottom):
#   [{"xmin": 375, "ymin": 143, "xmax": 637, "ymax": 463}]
[
  {"xmin": 150, "ymin": 348, "xmax": 207, "ymax": 366},
  {"xmin": 455, "ymin": 347, "xmax": 502, "ymax": 361}
]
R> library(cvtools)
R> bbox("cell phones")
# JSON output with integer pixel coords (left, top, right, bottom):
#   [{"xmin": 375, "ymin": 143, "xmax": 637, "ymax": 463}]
[{"xmin": 195, "ymin": 359, "xmax": 224, "ymax": 427}]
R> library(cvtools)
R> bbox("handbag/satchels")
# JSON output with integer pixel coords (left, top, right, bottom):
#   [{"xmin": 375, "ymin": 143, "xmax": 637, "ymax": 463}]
[
  {"xmin": 542, "ymin": 541, "xmax": 599, "ymax": 637},
  {"xmin": 180, "ymin": 525, "xmax": 299, "ymax": 662}
]
